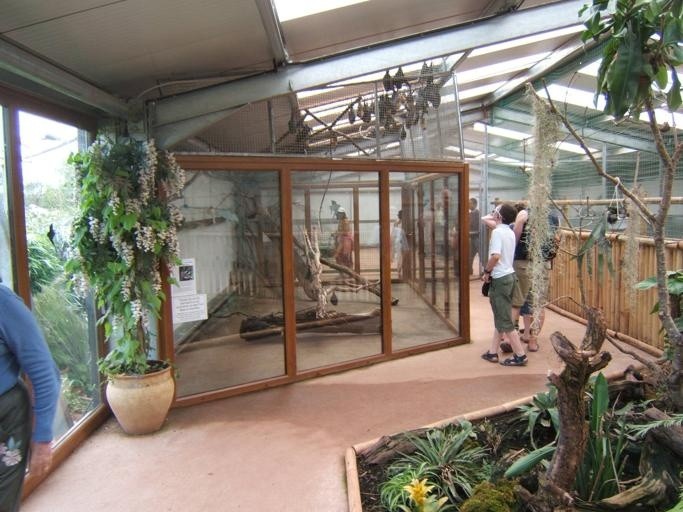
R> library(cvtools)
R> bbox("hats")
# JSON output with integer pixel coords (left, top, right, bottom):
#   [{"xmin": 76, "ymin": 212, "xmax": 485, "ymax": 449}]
[{"xmin": 336, "ymin": 207, "xmax": 346, "ymax": 214}]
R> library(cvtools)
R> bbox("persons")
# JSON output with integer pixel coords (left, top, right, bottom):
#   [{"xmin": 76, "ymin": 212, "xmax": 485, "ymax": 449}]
[
  {"xmin": 0, "ymin": 277, "xmax": 58, "ymax": 511},
  {"xmin": 333, "ymin": 227, "xmax": 354, "ymax": 279},
  {"xmin": 477, "ymin": 204, "xmax": 528, "ymax": 365},
  {"xmin": 331, "ymin": 210, "xmax": 352, "ymax": 269},
  {"xmin": 480, "ymin": 199, "xmax": 555, "ymax": 353},
  {"xmin": 389, "ymin": 209, "xmax": 402, "ymax": 276},
  {"xmin": 465, "ymin": 196, "xmax": 483, "ymax": 276}
]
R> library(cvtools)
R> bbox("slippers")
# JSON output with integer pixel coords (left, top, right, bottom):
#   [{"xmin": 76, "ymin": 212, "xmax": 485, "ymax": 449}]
[{"xmin": 500, "ymin": 326, "xmax": 539, "ymax": 352}]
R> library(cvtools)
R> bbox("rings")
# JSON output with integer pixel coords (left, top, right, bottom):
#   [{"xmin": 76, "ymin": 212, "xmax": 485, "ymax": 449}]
[{"xmin": 43, "ymin": 466, "xmax": 48, "ymax": 473}]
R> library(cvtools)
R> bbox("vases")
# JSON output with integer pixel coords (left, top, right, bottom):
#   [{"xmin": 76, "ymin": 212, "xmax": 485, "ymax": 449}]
[{"xmin": 104, "ymin": 359, "xmax": 175, "ymax": 434}]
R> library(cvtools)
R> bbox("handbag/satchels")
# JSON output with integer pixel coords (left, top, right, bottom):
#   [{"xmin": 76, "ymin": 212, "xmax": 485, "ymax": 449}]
[
  {"xmin": 328, "ymin": 249, "xmax": 337, "ymax": 256},
  {"xmin": 482, "ymin": 276, "xmax": 492, "ymax": 296}
]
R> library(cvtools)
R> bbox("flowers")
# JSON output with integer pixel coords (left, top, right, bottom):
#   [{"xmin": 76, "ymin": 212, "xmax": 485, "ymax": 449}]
[{"xmin": 57, "ymin": 128, "xmax": 183, "ymax": 372}]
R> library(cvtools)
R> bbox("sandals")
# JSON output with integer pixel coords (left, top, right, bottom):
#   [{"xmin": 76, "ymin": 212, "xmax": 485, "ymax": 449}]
[
  {"xmin": 481, "ymin": 350, "xmax": 499, "ymax": 362},
  {"xmin": 500, "ymin": 353, "xmax": 528, "ymax": 366}
]
